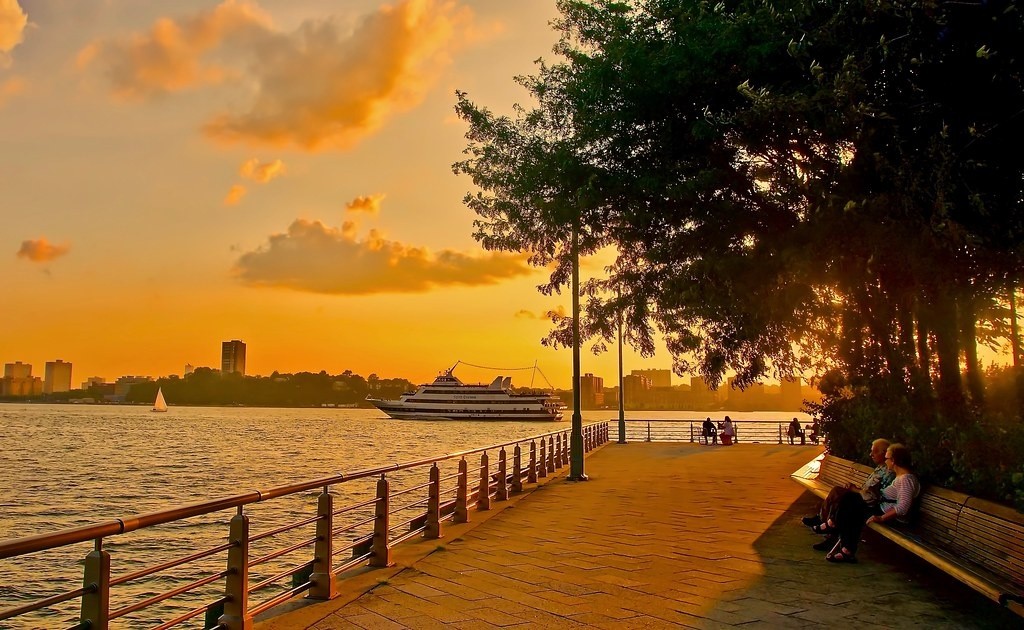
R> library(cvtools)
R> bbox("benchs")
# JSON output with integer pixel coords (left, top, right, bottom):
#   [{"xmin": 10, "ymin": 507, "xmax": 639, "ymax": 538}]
[{"xmin": 790, "ymin": 449, "xmax": 1024, "ymax": 606}]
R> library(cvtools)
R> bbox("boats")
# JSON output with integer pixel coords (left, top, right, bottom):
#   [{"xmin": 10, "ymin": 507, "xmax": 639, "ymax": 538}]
[{"xmin": 365, "ymin": 359, "xmax": 568, "ymax": 421}]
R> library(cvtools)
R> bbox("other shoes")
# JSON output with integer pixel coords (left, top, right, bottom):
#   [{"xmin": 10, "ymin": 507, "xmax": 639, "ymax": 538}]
[
  {"xmin": 813, "ymin": 536, "xmax": 839, "ymax": 551},
  {"xmin": 802, "ymin": 514, "xmax": 823, "ymax": 527}
]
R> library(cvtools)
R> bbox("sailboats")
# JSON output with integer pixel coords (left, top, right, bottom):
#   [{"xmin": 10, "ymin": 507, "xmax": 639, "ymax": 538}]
[{"xmin": 150, "ymin": 386, "xmax": 168, "ymax": 412}]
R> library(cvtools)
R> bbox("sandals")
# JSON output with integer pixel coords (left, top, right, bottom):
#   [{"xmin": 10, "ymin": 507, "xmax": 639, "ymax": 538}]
[
  {"xmin": 826, "ymin": 548, "xmax": 856, "ymax": 563},
  {"xmin": 812, "ymin": 522, "xmax": 836, "ymax": 534}
]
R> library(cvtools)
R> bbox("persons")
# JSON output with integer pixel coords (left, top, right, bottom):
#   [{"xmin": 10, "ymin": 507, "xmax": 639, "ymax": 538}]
[
  {"xmin": 721, "ymin": 416, "xmax": 733, "ymax": 444},
  {"xmin": 802, "ymin": 439, "xmax": 922, "ymax": 565},
  {"xmin": 788, "ymin": 418, "xmax": 806, "ymax": 445},
  {"xmin": 808, "ymin": 418, "xmax": 821, "ymax": 445},
  {"xmin": 703, "ymin": 417, "xmax": 718, "ymax": 446}
]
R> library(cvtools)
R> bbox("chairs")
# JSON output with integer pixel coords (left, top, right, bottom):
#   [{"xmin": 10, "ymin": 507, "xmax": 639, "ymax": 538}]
[{"xmin": 698, "ymin": 423, "xmax": 823, "ymax": 446}]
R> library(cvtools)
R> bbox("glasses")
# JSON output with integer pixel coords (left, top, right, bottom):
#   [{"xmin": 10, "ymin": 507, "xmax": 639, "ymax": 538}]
[{"xmin": 884, "ymin": 456, "xmax": 893, "ymax": 462}]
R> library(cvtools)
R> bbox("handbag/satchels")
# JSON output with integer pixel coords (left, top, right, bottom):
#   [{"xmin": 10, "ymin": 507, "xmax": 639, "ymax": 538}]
[{"xmin": 860, "ymin": 486, "xmax": 886, "ymax": 507}]
